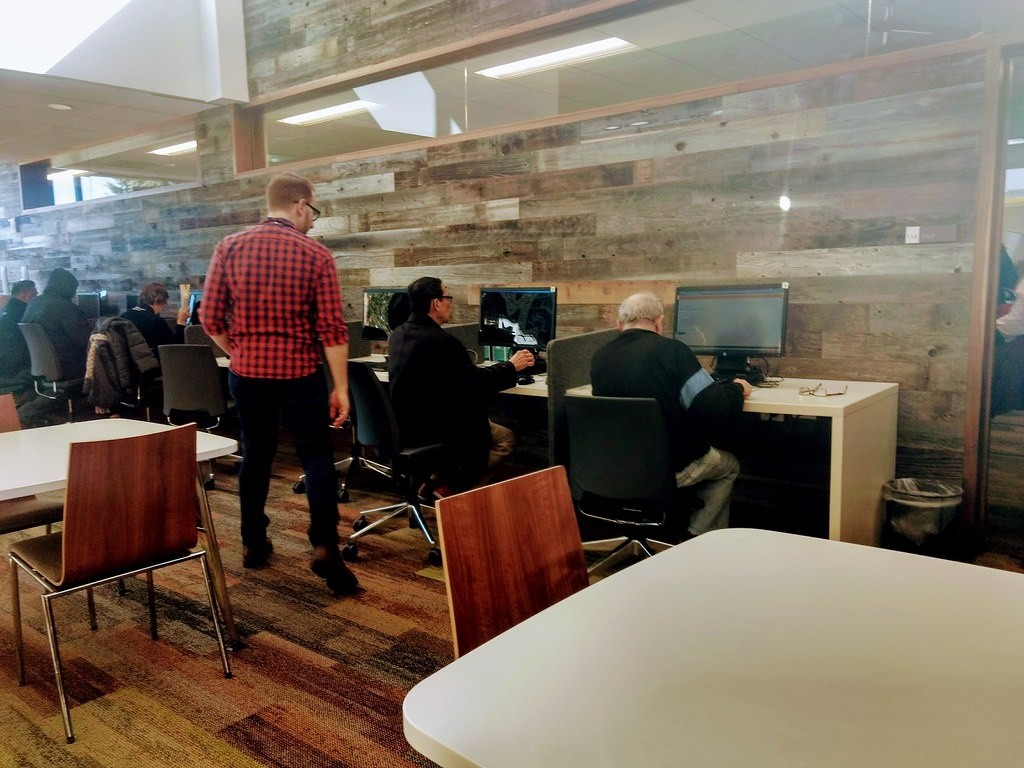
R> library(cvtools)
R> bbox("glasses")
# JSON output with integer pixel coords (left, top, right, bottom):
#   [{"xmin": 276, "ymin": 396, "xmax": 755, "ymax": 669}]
[
  {"xmin": 798, "ymin": 383, "xmax": 848, "ymax": 398},
  {"xmin": 434, "ymin": 294, "xmax": 454, "ymax": 305},
  {"xmin": 293, "ymin": 200, "xmax": 321, "ymax": 222}
]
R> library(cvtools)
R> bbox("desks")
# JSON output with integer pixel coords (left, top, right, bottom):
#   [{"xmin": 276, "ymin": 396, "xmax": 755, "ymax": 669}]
[
  {"xmin": 404, "ymin": 527, "xmax": 1024, "ymax": 768},
  {"xmin": 0, "ymin": 419, "xmax": 239, "ymax": 642},
  {"xmin": 215, "ymin": 352, "xmax": 898, "ymax": 546}
]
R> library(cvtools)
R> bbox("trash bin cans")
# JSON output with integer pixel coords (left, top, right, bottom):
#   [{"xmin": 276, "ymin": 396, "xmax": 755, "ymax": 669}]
[{"xmin": 884, "ymin": 478, "xmax": 963, "ymax": 561}]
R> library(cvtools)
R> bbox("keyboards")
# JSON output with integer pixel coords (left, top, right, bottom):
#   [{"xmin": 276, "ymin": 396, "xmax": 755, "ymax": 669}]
[{"xmin": 364, "ymin": 361, "xmax": 389, "ymax": 372}]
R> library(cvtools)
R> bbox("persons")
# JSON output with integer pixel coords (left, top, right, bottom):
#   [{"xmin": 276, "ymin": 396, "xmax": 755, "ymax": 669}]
[
  {"xmin": 994, "ymin": 280, "xmax": 1024, "ymax": 344},
  {"xmin": 588, "ymin": 291, "xmax": 751, "ymax": 540},
  {"xmin": 21, "ymin": 267, "xmax": 118, "ymax": 420},
  {"xmin": 116, "ymin": 280, "xmax": 191, "ymax": 384},
  {"xmin": 198, "ymin": 170, "xmax": 361, "ymax": 592},
  {"xmin": 386, "ymin": 276, "xmax": 535, "ymax": 499},
  {"xmin": 0, "ymin": 280, "xmax": 41, "ymax": 394}
]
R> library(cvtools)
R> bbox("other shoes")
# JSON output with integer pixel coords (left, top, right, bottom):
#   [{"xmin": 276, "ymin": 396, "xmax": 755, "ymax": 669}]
[
  {"xmin": 241, "ymin": 536, "xmax": 273, "ymax": 569},
  {"xmin": 417, "ymin": 476, "xmax": 437, "ymax": 501},
  {"xmin": 678, "ymin": 531, "xmax": 697, "ymax": 542},
  {"xmin": 309, "ymin": 545, "xmax": 359, "ymax": 594},
  {"xmin": 433, "ymin": 483, "xmax": 458, "ymax": 499}
]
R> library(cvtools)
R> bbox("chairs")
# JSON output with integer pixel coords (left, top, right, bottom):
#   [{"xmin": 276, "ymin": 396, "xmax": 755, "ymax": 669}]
[
  {"xmin": 7, "ymin": 422, "xmax": 232, "ymax": 744},
  {"xmin": 0, "ymin": 313, "xmax": 680, "ymax": 575},
  {"xmin": 435, "ymin": 466, "xmax": 590, "ymax": 661}
]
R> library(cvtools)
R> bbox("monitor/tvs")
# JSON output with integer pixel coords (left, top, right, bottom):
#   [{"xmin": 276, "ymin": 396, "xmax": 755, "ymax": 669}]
[
  {"xmin": 185, "ymin": 289, "xmax": 207, "ymax": 327},
  {"xmin": 479, "ymin": 287, "xmax": 557, "ymax": 375},
  {"xmin": 672, "ymin": 282, "xmax": 789, "ymax": 386},
  {"xmin": 361, "ymin": 287, "xmax": 409, "ymax": 340},
  {"xmin": 77, "ymin": 293, "xmax": 101, "ymax": 320}
]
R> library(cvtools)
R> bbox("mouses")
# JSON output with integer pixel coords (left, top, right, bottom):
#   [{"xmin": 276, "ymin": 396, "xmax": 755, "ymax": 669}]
[{"xmin": 518, "ymin": 377, "xmax": 534, "ymax": 385}]
